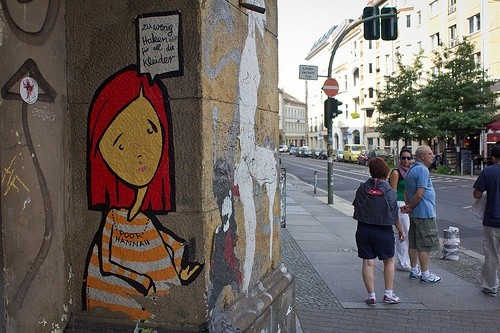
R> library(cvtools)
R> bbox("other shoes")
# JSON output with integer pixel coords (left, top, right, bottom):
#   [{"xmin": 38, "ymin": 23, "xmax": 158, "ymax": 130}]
[
  {"xmin": 397, "ymin": 263, "xmax": 419, "ymax": 272},
  {"xmin": 482, "ymin": 287, "xmax": 496, "ymax": 297}
]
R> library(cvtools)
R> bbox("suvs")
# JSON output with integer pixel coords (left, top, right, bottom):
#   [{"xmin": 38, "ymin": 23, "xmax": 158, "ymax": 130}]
[
  {"xmin": 279, "ymin": 145, "xmax": 288, "ymax": 153},
  {"xmin": 368, "ymin": 149, "xmax": 389, "ymax": 163},
  {"xmin": 358, "ymin": 150, "xmax": 369, "ymax": 166}
]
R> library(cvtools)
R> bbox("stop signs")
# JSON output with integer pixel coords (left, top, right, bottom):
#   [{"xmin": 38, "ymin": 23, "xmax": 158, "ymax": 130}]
[{"xmin": 323, "ymin": 78, "xmax": 339, "ymax": 96}]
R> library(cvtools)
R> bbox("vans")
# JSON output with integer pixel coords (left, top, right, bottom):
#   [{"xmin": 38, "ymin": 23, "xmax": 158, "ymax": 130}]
[{"xmin": 342, "ymin": 144, "xmax": 367, "ymax": 163}]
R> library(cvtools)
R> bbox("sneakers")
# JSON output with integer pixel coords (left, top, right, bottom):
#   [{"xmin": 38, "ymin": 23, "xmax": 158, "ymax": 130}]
[
  {"xmin": 383, "ymin": 294, "xmax": 401, "ymax": 304},
  {"xmin": 408, "ymin": 269, "xmax": 421, "ymax": 280},
  {"xmin": 365, "ymin": 297, "xmax": 377, "ymax": 306},
  {"xmin": 419, "ymin": 273, "xmax": 442, "ymax": 284}
]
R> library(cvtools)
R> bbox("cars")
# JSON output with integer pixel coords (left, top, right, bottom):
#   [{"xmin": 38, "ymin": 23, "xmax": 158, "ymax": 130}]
[
  {"xmin": 290, "ymin": 146, "xmax": 327, "ymax": 160},
  {"xmin": 334, "ymin": 149, "xmax": 343, "ymax": 161}
]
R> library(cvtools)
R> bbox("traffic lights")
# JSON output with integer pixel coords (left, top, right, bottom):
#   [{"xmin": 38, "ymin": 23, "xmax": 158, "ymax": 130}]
[{"xmin": 335, "ymin": 100, "xmax": 343, "ymax": 116}]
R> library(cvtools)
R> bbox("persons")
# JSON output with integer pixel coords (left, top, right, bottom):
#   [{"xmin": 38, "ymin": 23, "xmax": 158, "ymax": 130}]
[
  {"xmin": 473, "ymin": 145, "xmax": 500, "ymax": 296},
  {"xmin": 389, "ymin": 145, "xmax": 419, "ymax": 272},
  {"xmin": 400, "ymin": 146, "xmax": 442, "ymax": 284},
  {"xmin": 351, "ymin": 157, "xmax": 407, "ymax": 306}
]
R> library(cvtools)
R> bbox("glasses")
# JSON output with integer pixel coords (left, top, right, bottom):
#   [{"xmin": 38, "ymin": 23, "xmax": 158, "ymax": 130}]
[{"xmin": 399, "ymin": 156, "xmax": 413, "ymax": 160}]
